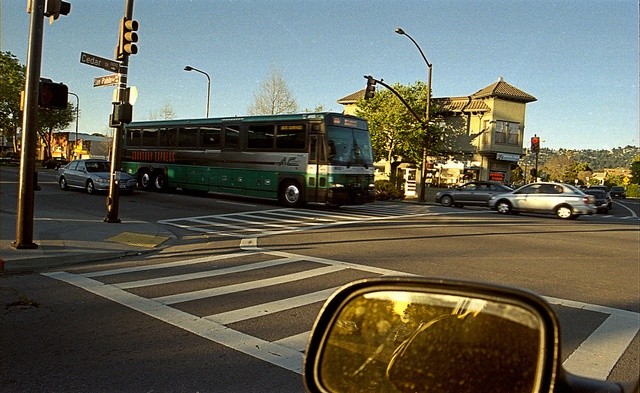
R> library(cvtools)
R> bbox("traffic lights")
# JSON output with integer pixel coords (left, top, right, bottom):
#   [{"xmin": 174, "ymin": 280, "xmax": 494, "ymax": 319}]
[
  {"xmin": 47, "ymin": 0, "xmax": 71, "ymax": 20},
  {"xmin": 120, "ymin": 17, "xmax": 139, "ymax": 54},
  {"xmin": 531, "ymin": 137, "xmax": 540, "ymax": 152},
  {"xmin": 39, "ymin": 82, "xmax": 68, "ymax": 109},
  {"xmin": 365, "ymin": 76, "xmax": 376, "ymax": 100}
]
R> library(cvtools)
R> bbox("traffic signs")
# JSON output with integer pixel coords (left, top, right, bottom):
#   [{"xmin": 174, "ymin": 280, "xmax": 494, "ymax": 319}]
[
  {"xmin": 94, "ymin": 74, "xmax": 119, "ymax": 86},
  {"xmin": 80, "ymin": 52, "xmax": 119, "ymax": 73}
]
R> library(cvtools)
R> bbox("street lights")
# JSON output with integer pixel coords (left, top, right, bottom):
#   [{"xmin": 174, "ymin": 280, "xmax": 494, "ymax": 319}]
[
  {"xmin": 395, "ymin": 27, "xmax": 432, "ymax": 202},
  {"xmin": 184, "ymin": 65, "xmax": 210, "ymax": 117}
]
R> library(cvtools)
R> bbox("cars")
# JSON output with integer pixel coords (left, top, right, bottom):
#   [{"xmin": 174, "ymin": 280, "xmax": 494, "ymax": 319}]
[
  {"xmin": 488, "ymin": 182, "xmax": 597, "ymax": 219},
  {"xmin": 584, "ymin": 189, "xmax": 608, "ymax": 214},
  {"xmin": 394, "ymin": 330, "xmax": 408, "ymax": 345},
  {"xmin": 610, "ymin": 186, "xmax": 626, "ymax": 199},
  {"xmin": 435, "ymin": 181, "xmax": 513, "ymax": 206},
  {"xmin": 56, "ymin": 159, "xmax": 136, "ymax": 194},
  {"xmin": 589, "ymin": 186, "xmax": 612, "ymax": 209},
  {"xmin": 42, "ymin": 157, "xmax": 69, "ymax": 169},
  {"xmin": 0, "ymin": 152, "xmax": 20, "ymax": 166}
]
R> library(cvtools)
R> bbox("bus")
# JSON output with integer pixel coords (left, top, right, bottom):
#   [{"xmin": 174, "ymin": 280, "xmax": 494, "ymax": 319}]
[{"xmin": 121, "ymin": 112, "xmax": 374, "ymax": 207}]
